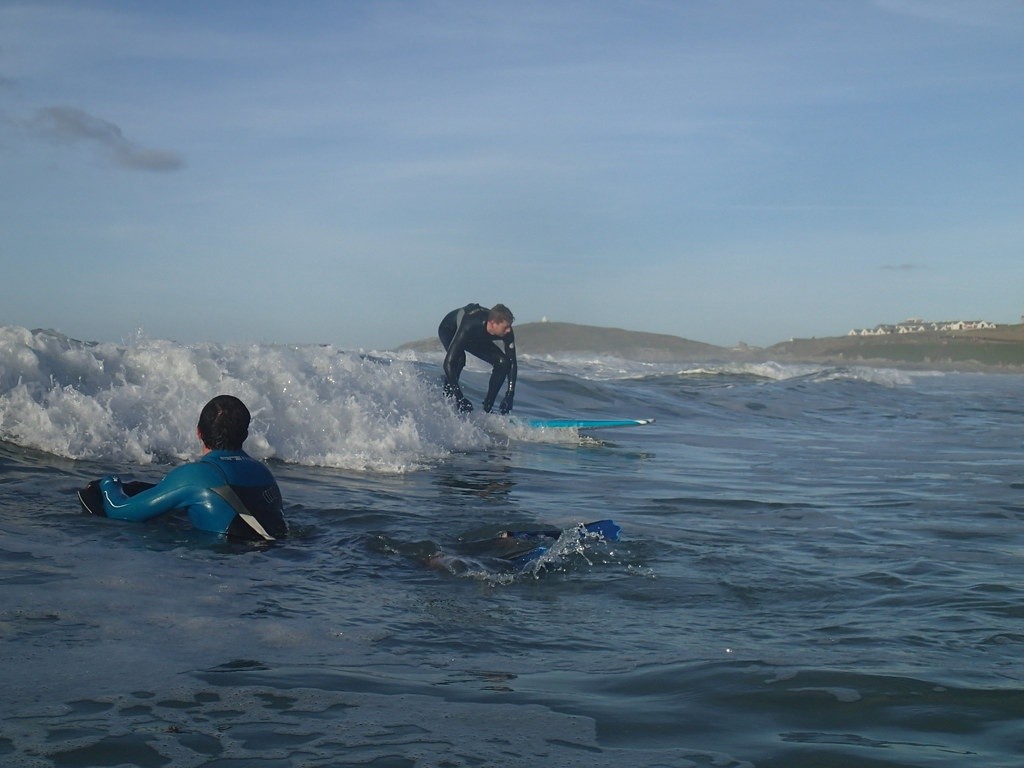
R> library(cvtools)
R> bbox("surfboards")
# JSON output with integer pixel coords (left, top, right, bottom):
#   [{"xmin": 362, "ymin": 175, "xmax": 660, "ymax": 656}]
[
  {"xmin": 523, "ymin": 416, "xmax": 654, "ymax": 430},
  {"xmin": 76, "ymin": 479, "xmax": 157, "ymax": 515}
]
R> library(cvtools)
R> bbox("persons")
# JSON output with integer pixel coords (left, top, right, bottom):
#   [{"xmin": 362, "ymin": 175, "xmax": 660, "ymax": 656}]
[
  {"xmin": 87, "ymin": 394, "xmax": 287, "ymax": 541},
  {"xmin": 438, "ymin": 303, "xmax": 518, "ymax": 414}
]
